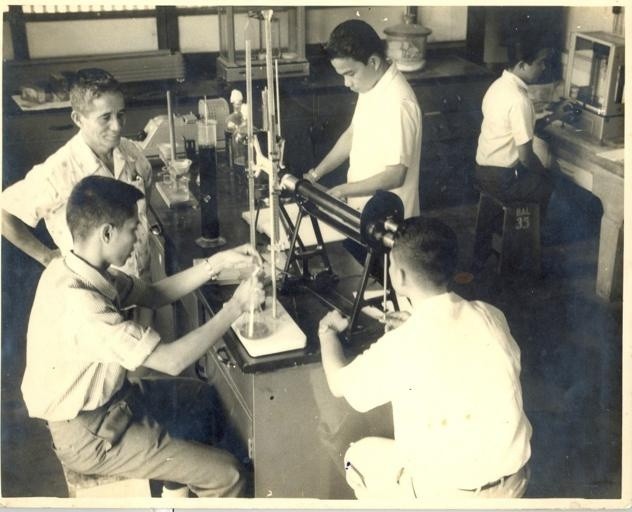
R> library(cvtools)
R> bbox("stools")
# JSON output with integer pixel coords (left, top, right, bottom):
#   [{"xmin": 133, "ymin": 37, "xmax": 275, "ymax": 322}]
[
  {"xmin": 68, "ymin": 454, "xmax": 152, "ymax": 499},
  {"xmin": 472, "ymin": 186, "xmax": 542, "ymax": 275}
]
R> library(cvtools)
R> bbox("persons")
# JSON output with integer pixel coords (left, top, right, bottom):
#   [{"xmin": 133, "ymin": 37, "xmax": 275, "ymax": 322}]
[
  {"xmin": 2, "ymin": 66, "xmax": 159, "ymax": 382},
  {"xmin": 472, "ymin": 33, "xmax": 591, "ymax": 281},
  {"xmin": 314, "ymin": 214, "xmax": 534, "ymax": 500},
  {"xmin": 298, "ymin": 17, "xmax": 426, "ymax": 225},
  {"xmin": 19, "ymin": 173, "xmax": 270, "ymax": 496}
]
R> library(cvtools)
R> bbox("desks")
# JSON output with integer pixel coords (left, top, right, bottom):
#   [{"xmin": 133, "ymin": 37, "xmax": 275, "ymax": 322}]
[
  {"xmin": 531, "ymin": 109, "xmax": 624, "ymax": 301},
  {"xmin": 3, "ymin": 53, "xmax": 497, "ymax": 214},
  {"xmin": 143, "ymin": 149, "xmax": 395, "ymax": 499}
]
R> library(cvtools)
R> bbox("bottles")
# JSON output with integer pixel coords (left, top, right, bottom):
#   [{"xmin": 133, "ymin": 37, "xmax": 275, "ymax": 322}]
[
  {"xmin": 239, "ymin": 303, "xmax": 272, "ymax": 340},
  {"xmin": 194, "ymin": 119, "xmax": 227, "ymax": 249},
  {"xmin": 224, "ymin": 102, "xmax": 261, "ymax": 186}
]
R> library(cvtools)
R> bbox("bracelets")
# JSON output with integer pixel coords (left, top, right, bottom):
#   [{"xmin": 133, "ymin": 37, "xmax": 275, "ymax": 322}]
[
  {"xmin": 315, "ymin": 324, "xmax": 340, "ymax": 336},
  {"xmin": 542, "ymin": 114, "xmax": 552, "ymax": 127},
  {"xmin": 308, "ymin": 167, "xmax": 320, "ymax": 181},
  {"xmin": 201, "ymin": 256, "xmax": 218, "ymax": 281}
]
